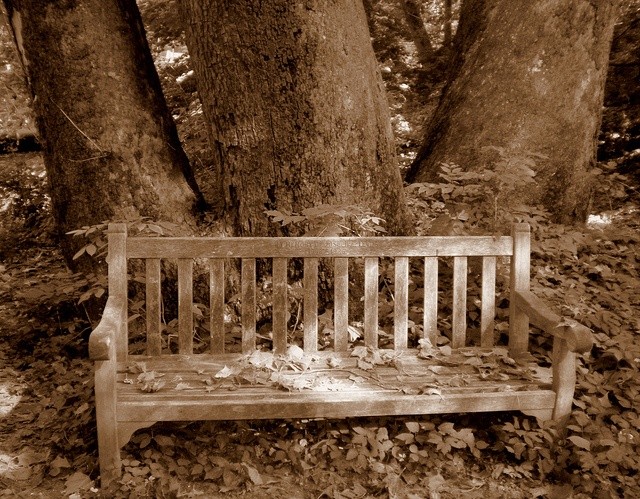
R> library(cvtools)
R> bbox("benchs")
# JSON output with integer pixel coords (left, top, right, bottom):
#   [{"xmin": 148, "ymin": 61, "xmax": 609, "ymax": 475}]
[{"xmin": 87, "ymin": 222, "xmax": 595, "ymax": 492}]
